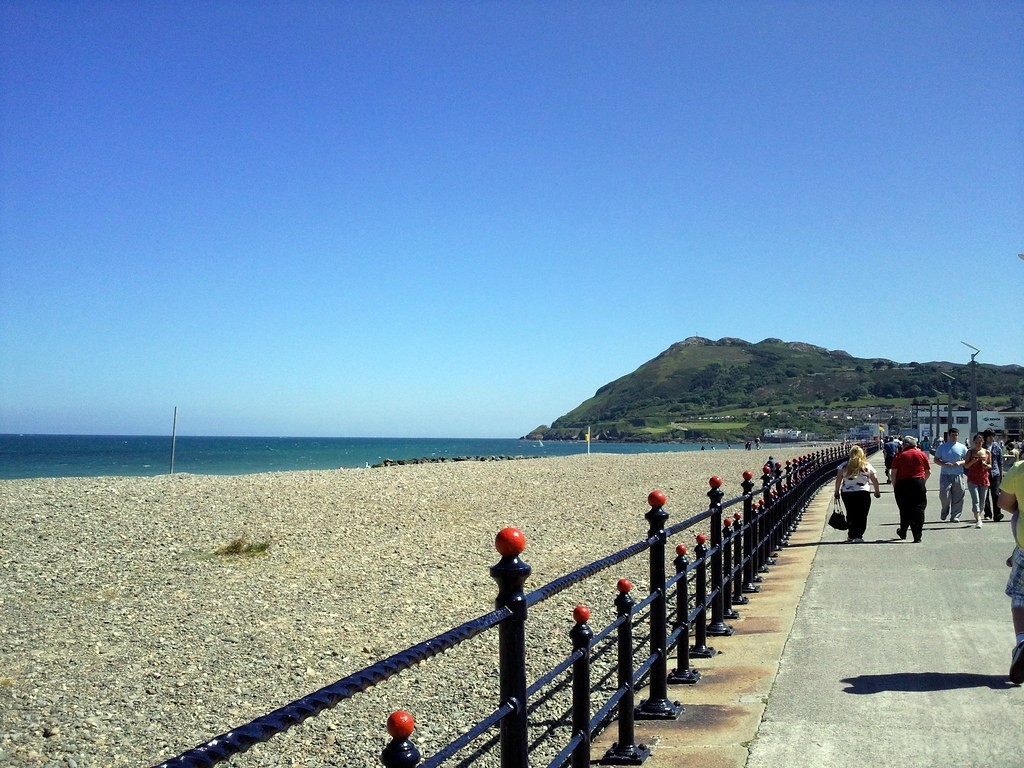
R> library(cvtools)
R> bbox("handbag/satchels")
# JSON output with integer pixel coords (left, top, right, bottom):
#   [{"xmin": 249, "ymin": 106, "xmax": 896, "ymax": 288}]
[
  {"xmin": 963, "ymin": 450, "xmax": 972, "ymax": 475},
  {"xmin": 828, "ymin": 495, "xmax": 848, "ymax": 530}
]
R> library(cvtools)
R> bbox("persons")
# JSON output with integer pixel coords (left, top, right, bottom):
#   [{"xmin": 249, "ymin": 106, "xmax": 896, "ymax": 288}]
[
  {"xmin": 980, "ymin": 427, "xmax": 1004, "ymax": 522},
  {"xmin": 701, "ymin": 435, "xmax": 763, "ymax": 450},
  {"xmin": 997, "ymin": 444, "xmax": 1024, "ymax": 686},
  {"xmin": 834, "ymin": 445, "xmax": 880, "ymax": 542},
  {"xmin": 764, "ymin": 455, "xmax": 776, "ymax": 478},
  {"xmin": 965, "ymin": 434, "xmax": 992, "ymax": 528},
  {"xmin": 879, "ymin": 433, "xmax": 1024, "ymax": 484},
  {"xmin": 1006, "ymin": 508, "xmax": 1019, "ymax": 567},
  {"xmin": 933, "ymin": 428, "xmax": 968, "ymax": 522},
  {"xmin": 891, "ymin": 435, "xmax": 931, "ymax": 543}
]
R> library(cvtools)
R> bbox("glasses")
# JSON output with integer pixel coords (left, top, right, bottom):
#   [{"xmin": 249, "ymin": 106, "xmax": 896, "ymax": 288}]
[{"xmin": 951, "ymin": 433, "xmax": 958, "ymax": 436}]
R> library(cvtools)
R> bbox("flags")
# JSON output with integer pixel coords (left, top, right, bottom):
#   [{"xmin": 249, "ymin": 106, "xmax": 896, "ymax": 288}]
[{"xmin": 879, "ymin": 423, "xmax": 885, "ymax": 431}]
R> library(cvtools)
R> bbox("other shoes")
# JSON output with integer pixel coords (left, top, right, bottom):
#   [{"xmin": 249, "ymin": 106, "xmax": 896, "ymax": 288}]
[
  {"xmin": 976, "ymin": 521, "xmax": 982, "ymax": 528},
  {"xmin": 887, "ymin": 480, "xmax": 892, "ymax": 484},
  {"xmin": 1006, "ymin": 557, "xmax": 1013, "ymax": 567},
  {"xmin": 855, "ymin": 538, "xmax": 864, "ymax": 542},
  {"xmin": 984, "ymin": 517, "xmax": 990, "ymax": 519},
  {"xmin": 914, "ymin": 538, "xmax": 921, "ymax": 543},
  {"xmin": 941, "ymin": 514, "xmax": 946, "ymax": 520},
  {"xmin": 844, "ymin": 537, "xmax": 855, "ymax": 543},
  {"xmin": 950, "ymin": 518, "xmax": 959, "ymax": 523},
  {"xmin": 1009, "ymin": 640, "xmax": 1024, "ymax": 684},
  {"xmin": 994, "ymin": 514, "xmax": 1004, "ymax": 521},
  {"xmin": 897, "ymin": 528, "xmax": 906, "ymax": 539}
]
